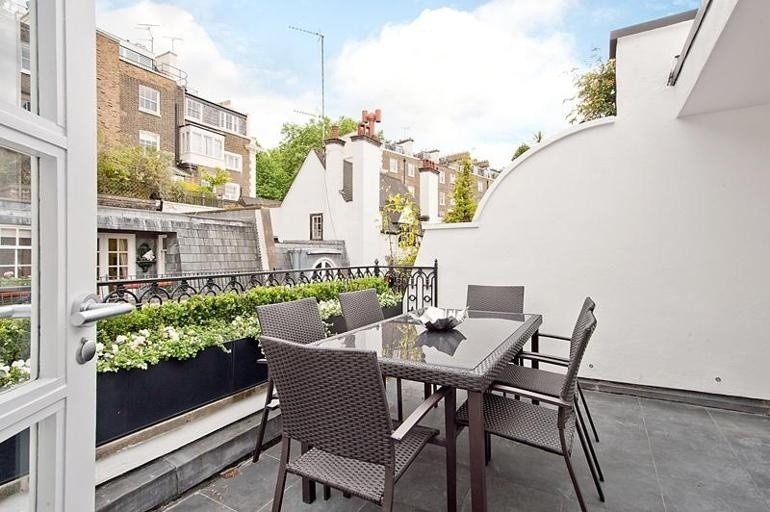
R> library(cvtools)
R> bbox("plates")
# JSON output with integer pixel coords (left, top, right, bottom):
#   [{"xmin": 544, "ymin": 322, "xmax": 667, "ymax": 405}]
[
  {"xmin": 412, "ymin": 327, "xmax": 468, "ymax": 357},
  {"xmin": 407, "ymin": 306, "xmax": 470, "ymax": 330}
]
